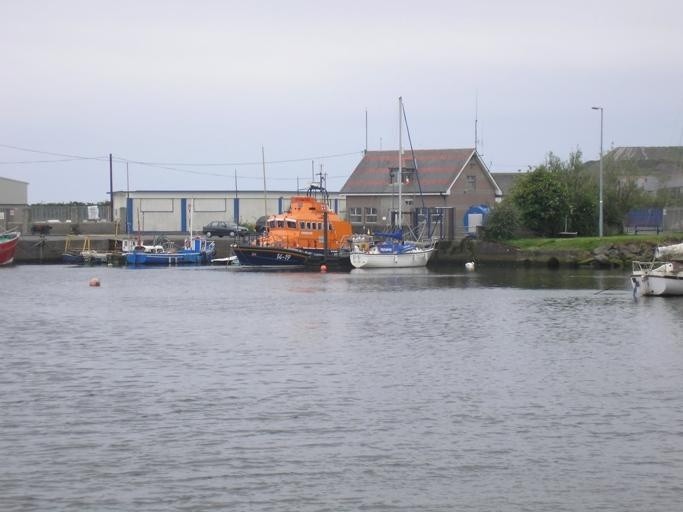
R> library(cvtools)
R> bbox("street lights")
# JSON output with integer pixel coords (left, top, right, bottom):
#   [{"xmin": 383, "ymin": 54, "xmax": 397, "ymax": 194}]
[{"xmin": 591, "ymin": 105, "xmax": 604, "ymax": 236}]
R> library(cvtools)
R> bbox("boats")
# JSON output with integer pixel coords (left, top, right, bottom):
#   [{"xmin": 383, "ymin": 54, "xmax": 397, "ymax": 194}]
[
  {"xmin": 629, "ymin": 243, "xmax": 683, "ymax": 302},
  {"xmin": 0, "ymin": 230, "xmax": 19, "ymax": 266}
]
